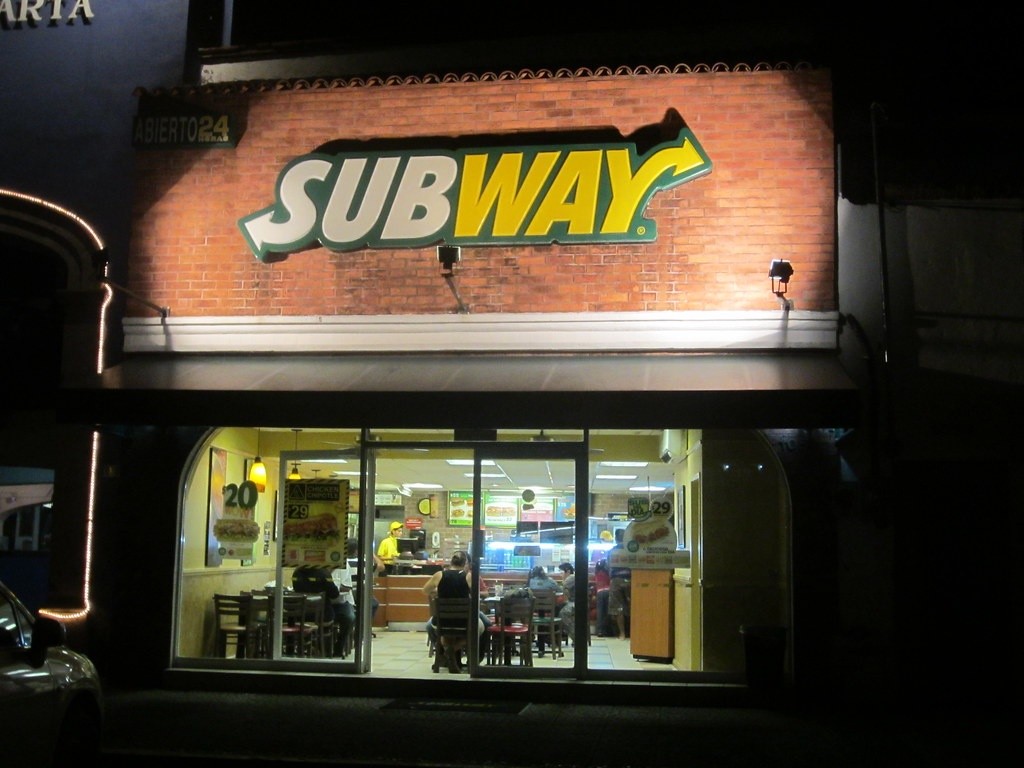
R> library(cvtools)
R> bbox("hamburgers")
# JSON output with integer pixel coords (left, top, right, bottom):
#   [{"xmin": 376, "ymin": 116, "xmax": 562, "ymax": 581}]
[
  {"xmin": 214, "ymin": 518, "xmax": 260, "ymax": 543},
  {"xmin": 283, "ymin": 513, "xmax": 340, "ymax": 548}
]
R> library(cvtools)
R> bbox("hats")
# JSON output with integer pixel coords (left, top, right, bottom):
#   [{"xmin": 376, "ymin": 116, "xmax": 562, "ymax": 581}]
[{"xmin": 390, "ymin": 522, "xmax": 403, "ymax": 531}]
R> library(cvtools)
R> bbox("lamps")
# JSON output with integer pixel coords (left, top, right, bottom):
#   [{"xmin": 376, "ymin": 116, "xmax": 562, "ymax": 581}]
[
  {"xmin": 289, "ymin": 429, "xmax": 303, "ymax": 479},
  {"xmin": 768, "ymin": 257, "xmax": 794, "ymax": 311},
  {"xmin": 249, "ymin": 427, "xmax": 267, "ymax": 492},
  {"xmin": 436, "ymin": 245, "xmax": 471, "ymax": 315}
]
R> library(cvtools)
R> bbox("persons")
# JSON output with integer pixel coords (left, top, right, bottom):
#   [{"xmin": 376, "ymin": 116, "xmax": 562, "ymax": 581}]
[
  {"xmin": 377, "ymin": 522, "xmax": 403, "ymax": 576},
  {"xmin": 292, "ymin": 539, "xmax": 385, "ymax": 660},
  {"xmin": 422, "ymin": 552, "xmax": 492, "ymax": 673},
  {"xmin": 559, "ymin": 563, "xmax": 575, "ymax": 625},
  {"xmin": 527, "ymin": 565, "xmax": 562, "ymax": 657},
  {"xmin": 595, "ymin": 559, "xmax": 631, "ymax": 640}
]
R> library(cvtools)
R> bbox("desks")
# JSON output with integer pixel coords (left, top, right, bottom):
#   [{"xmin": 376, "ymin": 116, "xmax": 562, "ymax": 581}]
[
  {"xmin": 479, "ymin": 596, "xmax": 499, "ymax": 624},
  {"xmin": 212, "ymin": 594, "xmax": 322, "ymax": 658}
]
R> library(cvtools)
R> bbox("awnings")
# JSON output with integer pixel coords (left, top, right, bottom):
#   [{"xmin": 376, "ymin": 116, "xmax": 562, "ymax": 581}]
[{"xmin": 53, "ymin": 357, "xmax": 859, "ymax": 426}]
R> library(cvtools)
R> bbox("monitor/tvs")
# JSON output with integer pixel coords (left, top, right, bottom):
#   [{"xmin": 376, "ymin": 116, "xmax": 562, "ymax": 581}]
[{"xmin": 397, "ymin": 537, "xmax": 419, "ymax": 555}]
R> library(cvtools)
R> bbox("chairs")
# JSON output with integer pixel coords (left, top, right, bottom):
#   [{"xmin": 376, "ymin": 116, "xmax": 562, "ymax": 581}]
[
  {"xmin": 214, "ymin": 586, "xmax": 355, "ymax": 659},
  {"xmin": 426, "ymin": 581, "xmax": 597, "ymax": 674}
]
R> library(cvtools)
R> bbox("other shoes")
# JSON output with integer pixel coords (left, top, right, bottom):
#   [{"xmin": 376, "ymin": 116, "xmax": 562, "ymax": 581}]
[
  {"xmin": 616, "ymin": 636, "xmax": 625, "ymax": 641},
  {"xmin": 598, "ymin": 632, "xmax": 603, "ymax": 637}
]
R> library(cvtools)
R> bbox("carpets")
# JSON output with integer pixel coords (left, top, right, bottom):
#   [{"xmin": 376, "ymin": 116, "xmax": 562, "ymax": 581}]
[{"xmin": 381, "ymin": 696, "xmax": 532, "ymax": 715}]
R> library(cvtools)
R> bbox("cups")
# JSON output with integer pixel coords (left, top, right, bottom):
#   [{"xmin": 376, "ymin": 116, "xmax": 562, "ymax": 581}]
[{"xmin": 494, "ymin": 584, "xmax": 503, "ymax": 597}]
